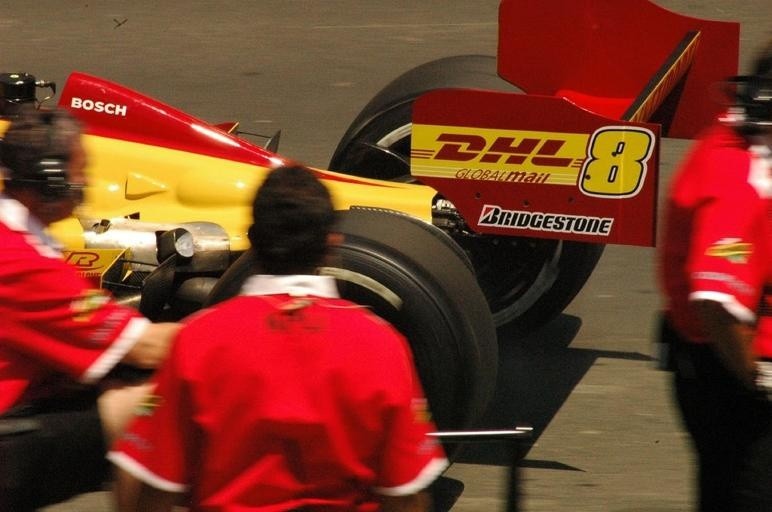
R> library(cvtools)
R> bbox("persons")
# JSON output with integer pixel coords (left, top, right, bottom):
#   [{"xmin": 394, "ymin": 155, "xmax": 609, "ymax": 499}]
[
  {"xmin": 649, "ymin": 44, "xmax": 772, "ymax": 511},
  {"xmin": 0, "ymin": 97, "xmax": 198, "ymax": 419},
  {"xmin": 105, "ymin": 164, "xmax": 464, "ymax": 512}
]
